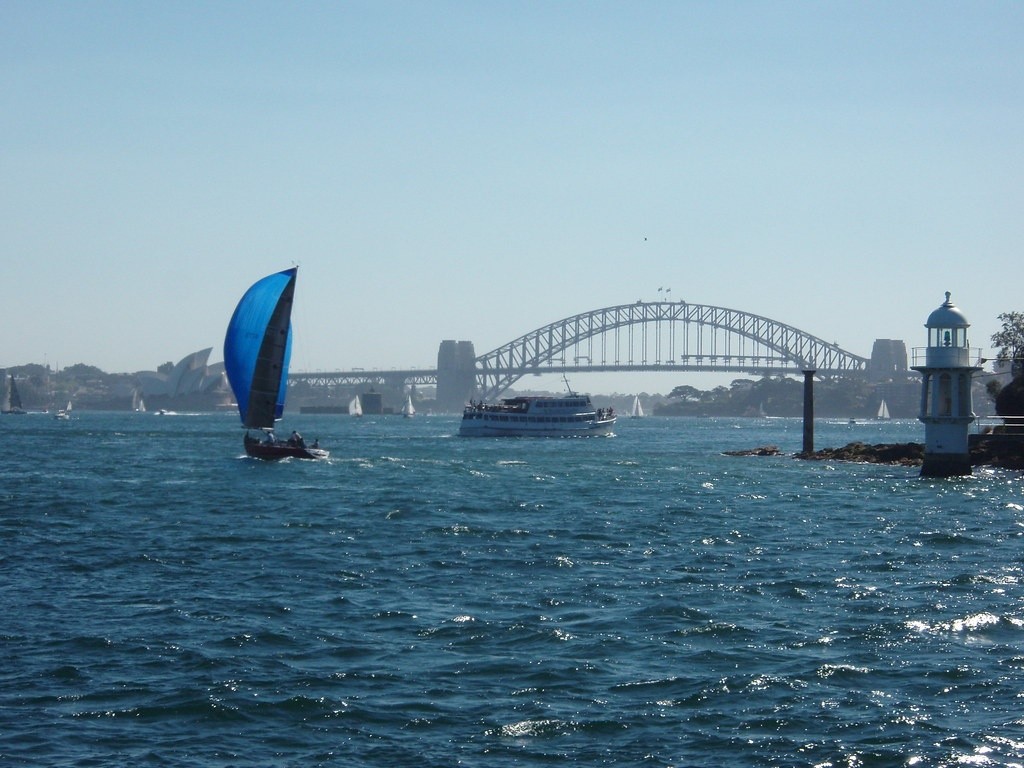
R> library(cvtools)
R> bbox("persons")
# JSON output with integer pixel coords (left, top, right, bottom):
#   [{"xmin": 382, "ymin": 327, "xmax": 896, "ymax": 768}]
[
  {"xmin": 264, "ymin": 431, "xmax": 275, "ymax": 445},
  {"xmin": 287, "ymin": 431, "xmax": 307, "ymax": 448},
  {"xmin": 596, "ymin": 406, "xmax": 614, "ymax": 421},
  {"xmin": 470, "ymin": 399, "xmax": 504, "ymax": 412},
  {"xmin": 311, "ymin": 439, "xmax": 319, "ymax": 449}
]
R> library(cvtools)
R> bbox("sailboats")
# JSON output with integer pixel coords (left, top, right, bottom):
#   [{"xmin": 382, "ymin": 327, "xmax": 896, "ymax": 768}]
[
  {"xmin": 878, "ymin": 399, "xmax": 891, "ymax": 420},
  {"xmin": 349, "ymin": 395, "xmax": 363, "ymax": 416},
  {"xmin": 0, "ymin": 375, "xmax": 28, "ymax": 415},
  {"xmin": 629, "ymin": 396, "xmax": 644, "ymax": 419},
  {"xmin": 401, "ymin": 395, "xmax": 416, "ymax": 418},
  {"xmin": 222, "ymin": 268, "xmax": 328, "ymax": 459}
]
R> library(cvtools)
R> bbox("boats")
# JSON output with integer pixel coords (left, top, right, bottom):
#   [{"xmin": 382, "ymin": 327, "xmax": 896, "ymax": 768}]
[
  {"xmin": 456, "ymin": 388, "xmax": 619, "ymax": 438},
  {"xmin": 54, "ymin": 410, "xmax": 70, "ymax": 420},
  {"xmin": 159, "ymin": 409, "xmax": 168, "ymax": 414},
  {"xmin": 849, "ymin": 417, "xmax": 855, "ymax": 424}
]
